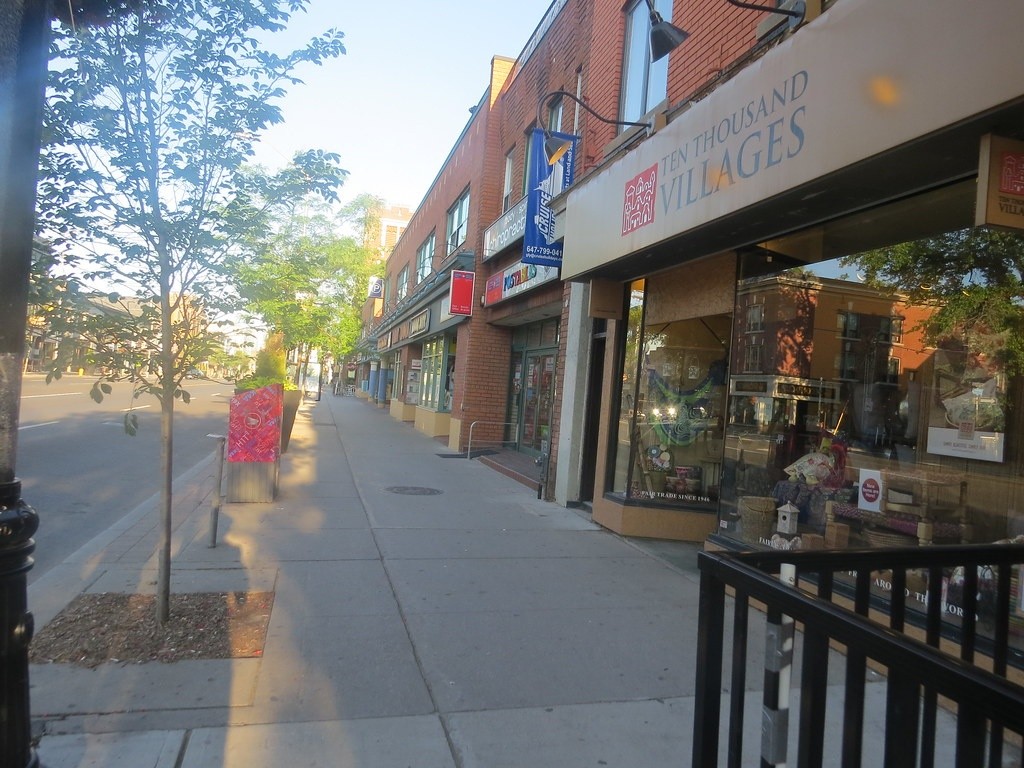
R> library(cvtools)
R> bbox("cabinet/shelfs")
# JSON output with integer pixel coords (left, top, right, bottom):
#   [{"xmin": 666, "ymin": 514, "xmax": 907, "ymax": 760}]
[{"xmin": 824, "ymin": 467, "xmax": 973, "ymax": 547}]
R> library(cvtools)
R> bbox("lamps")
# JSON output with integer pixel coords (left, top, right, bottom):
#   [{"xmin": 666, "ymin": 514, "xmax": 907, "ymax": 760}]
[
  {"xmin": 537, "ymin": 91, "xmax": 666, "ymax": 165},
  {"xmin": 646, "ymin": 0, "xmax": 806, "ymax": 64}
]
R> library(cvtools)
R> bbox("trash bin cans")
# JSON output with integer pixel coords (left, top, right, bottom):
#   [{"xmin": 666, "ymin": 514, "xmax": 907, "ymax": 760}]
[{"xmin": 225, "ymin": 383, "xmax": 285, "ymax": 504}]
